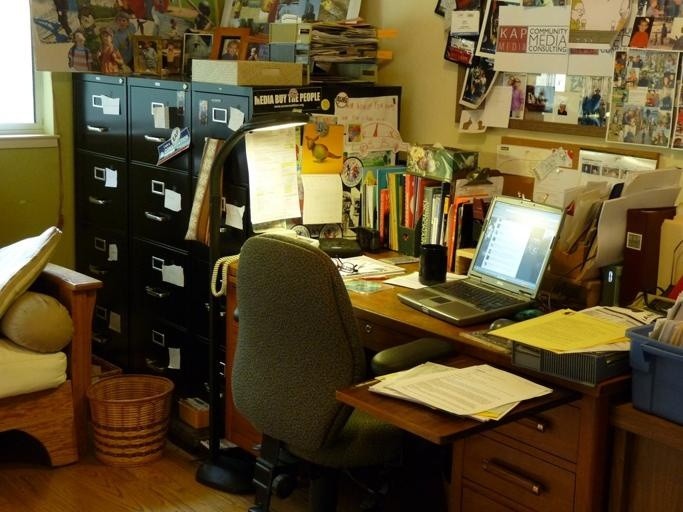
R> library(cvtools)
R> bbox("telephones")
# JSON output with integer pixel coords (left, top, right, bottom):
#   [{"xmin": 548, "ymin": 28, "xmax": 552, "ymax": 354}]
[{"xmin": 263, "ymin": 230, "xmax": 319, "ymax": 249}]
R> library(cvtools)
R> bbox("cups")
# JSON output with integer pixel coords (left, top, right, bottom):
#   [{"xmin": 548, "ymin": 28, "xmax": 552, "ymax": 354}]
[{"xmin": 418, "ymin": 243, "xmax": 449, "ymax": 286}]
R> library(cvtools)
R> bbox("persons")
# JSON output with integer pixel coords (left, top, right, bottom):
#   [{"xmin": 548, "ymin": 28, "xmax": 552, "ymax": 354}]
[
  {"xmin": 57, "ymin": 0, "xmax": 316, "ymax": 77},
  {"xmin": 471, "ymin": 0, "xmax": 682, "ymax": 149}
]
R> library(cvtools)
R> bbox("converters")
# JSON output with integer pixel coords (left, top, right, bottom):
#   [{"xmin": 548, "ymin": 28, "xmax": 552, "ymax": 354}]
[{"xmin": 648, "ymin": 298, "xmax": 675, "ymax": 315}]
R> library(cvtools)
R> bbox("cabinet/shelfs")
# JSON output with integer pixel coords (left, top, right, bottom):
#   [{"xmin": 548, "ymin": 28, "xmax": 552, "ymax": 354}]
[
  {"xmin": 72, "ymin": 72, "xmax": 402, "ymax": 465},
  {"xmin": 607, "ymin": 403, "xmax": 683, "ymax": 512}
]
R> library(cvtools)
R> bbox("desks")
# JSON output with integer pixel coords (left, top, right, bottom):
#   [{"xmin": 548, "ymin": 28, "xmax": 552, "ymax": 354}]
[{"xmin": 222, "ymin": 245, "xmax": 632, "ymax": 512}]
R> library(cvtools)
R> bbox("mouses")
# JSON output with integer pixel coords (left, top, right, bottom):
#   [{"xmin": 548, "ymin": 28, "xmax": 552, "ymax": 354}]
[{"xmin": 488, "ymin": 318, "xmax": 516, "ymax": 332}]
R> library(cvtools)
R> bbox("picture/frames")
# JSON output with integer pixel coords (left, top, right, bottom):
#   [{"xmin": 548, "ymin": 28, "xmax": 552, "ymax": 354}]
[
  {"xmin": 209, "ymin": 26, "xmax": 251, "ymax": 61},
  {"xmin": 131, "ymin": 34, "xmax": 163, "ymax": 77},
  {"xmin": 455, "ymin": 54, "xmax": 605, "ymax": 136}
]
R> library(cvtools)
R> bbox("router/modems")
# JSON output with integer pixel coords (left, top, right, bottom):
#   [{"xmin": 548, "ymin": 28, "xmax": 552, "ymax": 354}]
[{"xmin": 602, "ymin": 263, "xmax": 624, "ymax": 307}]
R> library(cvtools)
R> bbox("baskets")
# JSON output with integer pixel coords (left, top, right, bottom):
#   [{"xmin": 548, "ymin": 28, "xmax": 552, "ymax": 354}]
[{"xmin": 86, "ymin": 375, "xmax": 175, "ymax": 467}]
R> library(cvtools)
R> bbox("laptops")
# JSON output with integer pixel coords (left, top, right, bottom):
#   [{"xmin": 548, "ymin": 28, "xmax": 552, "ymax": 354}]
[{"xmin": 396, "ymin": 193, "xmax": 568, "ymax": 327}]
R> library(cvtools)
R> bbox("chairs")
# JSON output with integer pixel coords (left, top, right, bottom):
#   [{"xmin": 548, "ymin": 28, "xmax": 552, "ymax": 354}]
[
  {"xmin": 231, "ymin": 231, "xmax": 412, "ymax": 512},
  {"xmin": 0, "ymin": 261, "xmax": 104, "ymax": 470}
]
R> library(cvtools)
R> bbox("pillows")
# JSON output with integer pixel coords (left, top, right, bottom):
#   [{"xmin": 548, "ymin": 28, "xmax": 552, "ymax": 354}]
[
  {"xmin": 0, "ymin": 225, "xmax": 63, "ymax": 321},
  {"xmin": 1, "ymin": 291, "xmax": 76, "ymax": 354}
]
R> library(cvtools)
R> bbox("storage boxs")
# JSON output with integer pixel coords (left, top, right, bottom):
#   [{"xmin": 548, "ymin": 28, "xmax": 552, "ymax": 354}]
[{"xmin": 625, "ymin": 323, "xmax": 682, "ymax": 426}]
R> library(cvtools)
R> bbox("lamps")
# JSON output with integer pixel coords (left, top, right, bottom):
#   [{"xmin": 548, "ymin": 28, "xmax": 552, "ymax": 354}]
[{"xmin": 199, "ymin": 112, "xmax": 309, "ymax": 496}]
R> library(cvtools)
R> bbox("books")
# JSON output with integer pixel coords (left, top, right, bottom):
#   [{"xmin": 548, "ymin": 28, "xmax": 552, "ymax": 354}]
[{"xmin": 359, "ymin": 165, "xmax": 505, "ymax": 275}]
